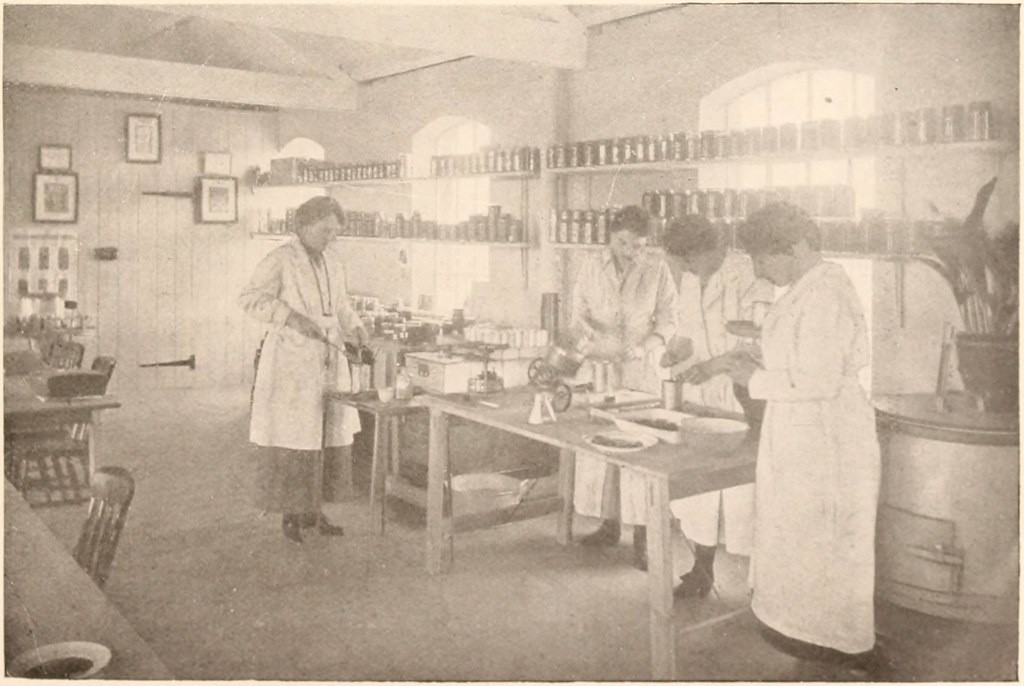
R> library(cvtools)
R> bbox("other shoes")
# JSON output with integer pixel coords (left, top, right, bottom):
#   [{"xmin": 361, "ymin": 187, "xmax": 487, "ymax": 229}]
[
  {"xmin": 581, "ymin": 520, "xmax": 621, "ymax": 545},
  {"xmin": 633, "ymin": 525, "xmax": 647, "ymax": 569},
  {"xmin": 283, "ymin": 516, "xmax": 305, "ymax": 545},
  {"xmin": 302, "ymin": 512, "xmax": 343, "ymax": 536}
]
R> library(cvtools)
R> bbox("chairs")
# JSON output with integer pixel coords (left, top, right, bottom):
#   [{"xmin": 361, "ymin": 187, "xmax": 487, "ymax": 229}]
[
  {"xmin": 4, "ymin": 338, "xmax": 118, "ymax": 509},
  {"xmin": 72, "ymin": 465, "xmax": 136, "ymax": 585}
]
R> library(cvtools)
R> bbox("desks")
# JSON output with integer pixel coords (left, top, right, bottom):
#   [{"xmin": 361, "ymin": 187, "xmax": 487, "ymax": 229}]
[
  {"xmin": 429, "ymin": 382, "xmax": 761, "ymax": 683},
  {"xmin": 323, "ymin": 390, "xmax": 452, "ymax": 534},
  {"xmin": 4, "ymin": 477, "xmax": 177, "ymax": 680},
  {"xmin": 3, "ymin": 360, "xmax": 121, "ymax": 417}
]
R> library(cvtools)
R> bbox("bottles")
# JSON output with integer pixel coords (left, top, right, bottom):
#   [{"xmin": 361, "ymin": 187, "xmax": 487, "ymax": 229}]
[
  {"xmin": 250, "ymin": 103, "xmax": 990, "ymax": 258},
  {"xmin": 346, "ymin": 293, "xmax": 621, "ymax": 401}
]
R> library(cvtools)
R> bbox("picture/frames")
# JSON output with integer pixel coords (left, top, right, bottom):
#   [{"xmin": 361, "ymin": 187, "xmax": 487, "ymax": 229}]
[
  {"xmin": 126, "ymin": 113, "xmax": 162, "ymax": 164},
  {"xmin": 32, "ymin": 171, "xmax": 80, "ymax": 223},
  {"xmin": 195, "ymin": 174, "xmax": 239, "ymax": 224},
  {"xmin": 37, "ymin": 144, "xmax": 72, "ymax": 170},
  {"xmin": 205, "ymin": 150, "xmax": 232, "ymax": 174}
]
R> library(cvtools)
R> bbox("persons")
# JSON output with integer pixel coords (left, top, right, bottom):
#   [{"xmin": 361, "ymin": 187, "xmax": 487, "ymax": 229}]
[
  {"xmin": 720, "ymin": 199, "xmax": 881, "ymax": 667},
  {"xmin": 569, "ymin": 205, "xmax": 680, "ymax": 571},
  {"xmin": 660, "ymin": 216, "xmax": 775, "ymax": 596},
  {"xmin": 240, "ymin": 196, "xmax": 369, "ymax": 541}
]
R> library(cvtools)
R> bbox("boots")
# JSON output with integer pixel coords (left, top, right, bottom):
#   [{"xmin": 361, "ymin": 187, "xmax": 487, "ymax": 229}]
[{"xmin": 673, "ymin": 544, "xmax": 713, "ymax": 597}]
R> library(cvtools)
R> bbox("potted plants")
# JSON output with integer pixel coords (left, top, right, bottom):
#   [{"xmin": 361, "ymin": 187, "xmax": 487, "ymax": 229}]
[{"xmin": 880, "ymin": 178, "xmax": 1020, "ymax": 411}]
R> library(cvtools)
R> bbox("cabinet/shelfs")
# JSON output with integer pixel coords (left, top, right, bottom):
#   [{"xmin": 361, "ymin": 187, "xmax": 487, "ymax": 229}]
[
  {"xmin": 550, "ymin": 141, "xmax": 1019, "ymax": 261},
  {"xmin": 251, "ymin": 170, "xmax": 542, "ymax": 251}
]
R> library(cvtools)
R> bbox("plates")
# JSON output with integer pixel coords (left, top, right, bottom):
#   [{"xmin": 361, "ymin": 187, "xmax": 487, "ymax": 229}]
[
  {"xmin": 7, "ymin": 640, "xmax": 111, "ymax": 679},
  {"xmin": 584, "ymin": 409, "xmax": 693, "ymax": 454}
]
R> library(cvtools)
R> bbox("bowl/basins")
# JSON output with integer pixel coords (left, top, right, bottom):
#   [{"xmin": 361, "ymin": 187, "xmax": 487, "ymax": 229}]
[{"xmin": 678, "ymin": 418, "xmax": 750, "ymax": 457}]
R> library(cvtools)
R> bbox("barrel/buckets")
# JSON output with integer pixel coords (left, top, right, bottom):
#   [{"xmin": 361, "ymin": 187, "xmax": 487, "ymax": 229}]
[{"xmin": 448, "ymin": 473, "xmax": 525, "ymax": 520}]
